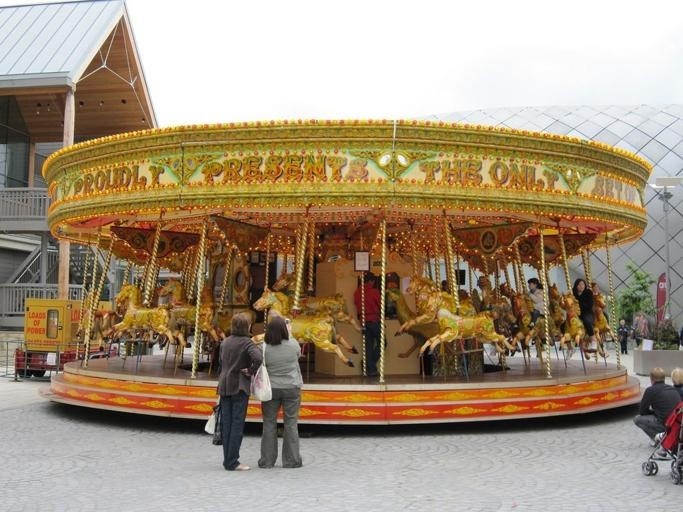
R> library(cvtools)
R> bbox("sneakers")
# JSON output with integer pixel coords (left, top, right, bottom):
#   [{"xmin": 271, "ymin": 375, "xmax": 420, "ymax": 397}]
[
  {"xmin": 361, "ymin": 361, "xmax": 379, "ymax": 376},
  {"xmin": 587, "ymin": 342, "xmax": 598, "ymax": 352}
]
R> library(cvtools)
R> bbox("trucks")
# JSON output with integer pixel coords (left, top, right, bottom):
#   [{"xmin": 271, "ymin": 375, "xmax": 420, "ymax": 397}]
[{"xmin": 15, "ymin": 297, "xmax": 121, "ymax": 380}]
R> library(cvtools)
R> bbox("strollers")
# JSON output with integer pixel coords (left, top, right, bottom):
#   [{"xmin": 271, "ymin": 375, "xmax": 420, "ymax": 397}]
[{"xmin": 641, "ymin": 400, "xmax": 683, "ymax": 486}]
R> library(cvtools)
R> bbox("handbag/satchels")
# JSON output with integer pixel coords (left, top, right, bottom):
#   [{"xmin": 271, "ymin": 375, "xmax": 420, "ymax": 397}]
[
  {"xmin": 211, "ymin": 402, "xmax": 224, "ymax": 445},
  {"xmin": 203, "ymin": 394, "xmax": 219, "ymax": 434},
  {"xmin": 249, "ymin": 342, "xmax": 273, "ymax": 403}
]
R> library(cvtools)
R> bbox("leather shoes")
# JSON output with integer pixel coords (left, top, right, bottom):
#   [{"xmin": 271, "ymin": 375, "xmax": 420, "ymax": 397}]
[{"xmin": 235, "ymin": 463, "xmax": 252, "ymax": 470}]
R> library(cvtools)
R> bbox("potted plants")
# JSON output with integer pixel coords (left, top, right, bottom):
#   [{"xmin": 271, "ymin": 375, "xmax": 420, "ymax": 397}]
[{"xmin": 615, "ymin": 264, "xmax": 683, "ymax": 375}]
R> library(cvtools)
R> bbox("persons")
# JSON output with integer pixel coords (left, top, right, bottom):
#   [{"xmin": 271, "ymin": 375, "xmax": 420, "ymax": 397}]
[
  {"xmin": 616, "ymin": 318, "xmax": 629, "ymax": 355},
  {"xmin": 215, "ymin": 311, "xmax": 264, "ymax": 471},
  {"xmin": 353, "ymin": 271, "xmax": 388, "ymax": 378},
  {"xmin": 632, "ymin": 366, "xmax": 682, "ymax": 445},
  {"xmin": 525, "ymin": 277, "xmax": 609, "ymax": 352},
  {"xmin": 257, "ymin": 315, "xmax": 304, "ymax": 469},
  {"xmin": 668, "ymin": 366, "xmax": 683, "ymax": 403},
  {"xmin": 640, "ymin": 313, "xmax": 650, "ymax": 339},
  {"xmin": 630, "ymin": 313, "xmax": 640, "ymax": 340}
]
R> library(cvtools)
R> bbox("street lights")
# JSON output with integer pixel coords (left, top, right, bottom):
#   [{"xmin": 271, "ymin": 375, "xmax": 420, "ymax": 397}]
[{"xmin": 649, "ymin": 190, "xmax": 682, "ymax": 327}]
